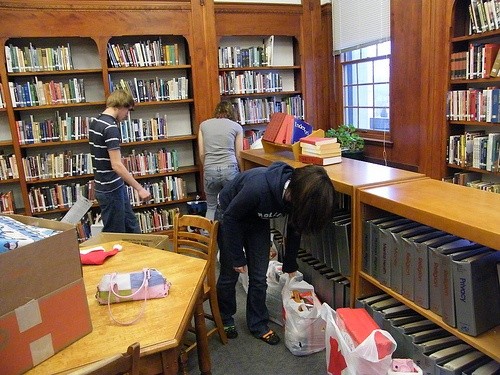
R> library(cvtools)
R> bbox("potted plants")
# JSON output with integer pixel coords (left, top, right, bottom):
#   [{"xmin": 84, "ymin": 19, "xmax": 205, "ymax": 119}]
[{"xmin": 325, "ymin": 124, "xmax": 364, "ymax": 156}]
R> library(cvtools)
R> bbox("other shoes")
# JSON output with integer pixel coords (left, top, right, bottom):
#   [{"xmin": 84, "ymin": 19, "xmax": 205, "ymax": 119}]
[
  {"xmin": 223, "ymin": 324, "xmax": 238, "ymax": 338},
  {"xmin": 262, "ymin": 329, "xmax": 280, "ymax": 345}
]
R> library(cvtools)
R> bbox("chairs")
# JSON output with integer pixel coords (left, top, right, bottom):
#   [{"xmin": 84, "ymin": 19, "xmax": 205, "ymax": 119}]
[{"xmin": 174, "ymin": 213, "xmax": 229, "ymax": 375}]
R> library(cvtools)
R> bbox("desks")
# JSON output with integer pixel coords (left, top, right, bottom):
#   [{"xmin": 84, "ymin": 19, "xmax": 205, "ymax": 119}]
[{"xmin": 24, "ymin": 240, "xmax": 209, "ymax": 375}]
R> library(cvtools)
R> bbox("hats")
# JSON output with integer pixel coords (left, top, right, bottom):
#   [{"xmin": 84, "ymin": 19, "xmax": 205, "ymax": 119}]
[{"xmin": 80, "ymin": 244, "xmax": 123, "ymax": 265}]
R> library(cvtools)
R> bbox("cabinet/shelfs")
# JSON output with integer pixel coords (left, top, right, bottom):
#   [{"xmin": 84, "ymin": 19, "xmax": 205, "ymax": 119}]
[
  {"xmin": 440, "ymin": 0, "xmax": 500, "ymax": 193},
  {"xmin": 357, "ymin": 177, "xmax": 500, "ymax": 375},
  {"xmin": 236, "ymin": 149, "xmax": 426, "ymax": 309},
  {"xmin": 0, "ymin": 0, "xmax": 328, "ymax": 238}
]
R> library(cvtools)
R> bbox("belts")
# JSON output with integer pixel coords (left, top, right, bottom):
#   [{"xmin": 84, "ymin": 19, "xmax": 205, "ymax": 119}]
[{"xmin": 204, "ymin": 166, "xmax": 239, "ymax": 171}]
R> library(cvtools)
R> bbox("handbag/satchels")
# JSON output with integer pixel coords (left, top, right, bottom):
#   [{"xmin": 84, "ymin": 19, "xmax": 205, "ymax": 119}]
[
  {"xmin": 281, "ymin": 276, "xmax": 326, "ymax": 356},
  {"xmin": 265, "ymin": 260, "xmax": 304, "ymax": 327},
  {"xmin": 387, "ymin": 358, "xmax": 423, "ymax": 375},
  {"xmin": 238, "ymin": 233, "xmax": 278, "ymax": 294},
  {"xmin": 95, "ymin": 267, "xmax": 171, "ymax": 326},
  {"xmin": 319, "ymin": 302, "xmax": 397, "ymax": 375}
]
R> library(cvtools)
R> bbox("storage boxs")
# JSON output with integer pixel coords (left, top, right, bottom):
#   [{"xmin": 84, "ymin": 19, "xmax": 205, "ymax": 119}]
[{"xmin": 0, "ymin": 213, "xmax": 92, "ymax": 375}]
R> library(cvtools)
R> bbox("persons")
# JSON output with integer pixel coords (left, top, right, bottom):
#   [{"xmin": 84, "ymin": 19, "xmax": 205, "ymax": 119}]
[
  {"xmin": 89, "ymin": 90, "xmax": 151, "ymax": 233},
  {"xmin": 198, "ymin": 101, "xmax": 244, "ymax": 254},
  {"xmin": 215, "ymin": 162, "xmax": 335, "ymax": 345}
]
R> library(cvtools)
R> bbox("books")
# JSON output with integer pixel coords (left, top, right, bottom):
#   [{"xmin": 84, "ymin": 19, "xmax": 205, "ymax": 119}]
[
  {"xmin": 219, "ymin": 71, "xmax": 282, "ymax": 95},
  {"xmin": 233, "ymin": 95, "xmax": 304, "ymax": 125},
  {"xmin": 108, "ymin": 37, "xmax": 179, "ymax": 68},
  {"xmin": 442, "ymin": 171, "xmax": 500, "ymax": 194},
  {"xmin": 299, "ymin": 138, "xmax": 342, "ymax": 166},
  {"xmin": 218, "ymin": 36, "xmax": 274, "ymax": 68},
  {"xmin": 243, "ymin": 129, "xmax": 266, "ymax": 150},
  {"xmin": 451, "ymin": 43, "xmax": 500, "ymax": 79},
  {"xmin": 0, "ymin": 44, "xmax": 85, "ymax": 108},
  {"xmin": 121, "ymin": 149, "xmax": 186, "ymax": 234},
  {"xmin": 469, "ymin": 0, "xmax": 500, "ymax": 35},
  {"xmin": 16, "ymin": 111, "xmax": 98, "ymax": 145},
  {"xmin": 118, "ymin": 111, "xmax": 168, "ymax": 143},
  {"xmin": 446, "ymin": 130, "xmax": 500, "ymax": 172},
  {"xmin": 109, "ymin": 74, "xmax": 188, "ymax": 103},
  {"xmin": 0, "ymin": 150, "xmax": 103, "ymax": 244},
  {"xmin": 446, "ymin": 87, "xmax": 500, "ymax": 123}
]
